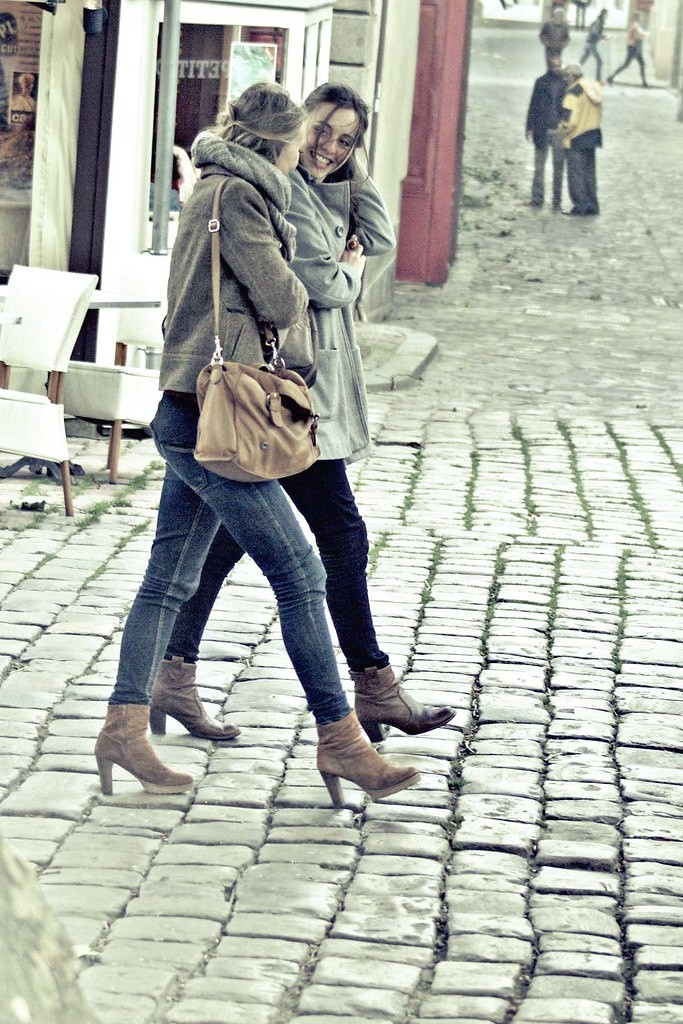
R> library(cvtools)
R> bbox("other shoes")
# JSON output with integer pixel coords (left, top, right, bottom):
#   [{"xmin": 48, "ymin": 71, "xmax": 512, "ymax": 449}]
[
  {"xmin": 551, "ymin": 205, "xmax": 560, "ymax": 212},
  {"xmin": 523, "ymin": 201, "xmax": 540, "ymax": 206},
  {"xmin": 562, "ymin": 209, "xmax": 585, "ymax": 216}
]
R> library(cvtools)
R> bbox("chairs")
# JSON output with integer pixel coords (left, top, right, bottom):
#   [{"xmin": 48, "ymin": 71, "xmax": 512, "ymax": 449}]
[
  {"xmin": 1, "ymin": 263, "xmax": 99, "ymax": 517},
  {"xmin": 58, "ymin": 302, "xmax": 170, "ymax": 485}
]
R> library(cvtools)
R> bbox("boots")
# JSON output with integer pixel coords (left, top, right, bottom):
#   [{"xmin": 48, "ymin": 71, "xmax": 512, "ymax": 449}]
[
  {"xmin": 317, "ymin": 709, "xmax": 421, "ymax": 807},
  {"xmin": 348, "ymin": 663, "xmax": 457, "ymax": 743},
  {"xmin": 95, "ymin": 704, "xmax": 194, "ymax": 795},
  {"xmin": 150, "ymin": 656, "xmax": 241, "ymax": 739}
]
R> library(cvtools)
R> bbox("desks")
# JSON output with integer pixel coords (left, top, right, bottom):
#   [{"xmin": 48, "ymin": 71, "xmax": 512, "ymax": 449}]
[{"xmin": 0, "ymin": 284, "xmax": 161, "ymax": 486}]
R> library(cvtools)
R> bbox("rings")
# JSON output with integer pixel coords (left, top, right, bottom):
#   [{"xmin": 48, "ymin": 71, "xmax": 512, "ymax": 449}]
[{"xmin": 347, "ymin": 239, "xmax": 358, "ymax": 252}]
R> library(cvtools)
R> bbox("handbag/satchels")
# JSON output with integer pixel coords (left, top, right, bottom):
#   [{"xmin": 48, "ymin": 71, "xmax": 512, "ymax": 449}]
[{"xmin": 193, "ymin": 361, "xmax": 321, "ymax": 481}]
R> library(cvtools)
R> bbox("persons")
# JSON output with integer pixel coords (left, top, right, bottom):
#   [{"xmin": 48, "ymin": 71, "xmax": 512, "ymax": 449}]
[
  {"xmin": 550, "ymin": 0, "xmax": 591, "ymax": 33},
  {"xmin": 93, "ymin": 82, "xmax": 455, "ymax": 806},
  {"xmin": 539, "ymin": 8, "xmax": 570, "ymax": 59},
  {"xmin": 522, "ymin": 55, "xmax": 602, "ymax": 215},
  {"xmin": 607, "ymin": 11, "xmax": 650, "ymax": 89},
  {"xmin": 579, "ymin": 9, "xmax": 609, "ymax": 87}
]
